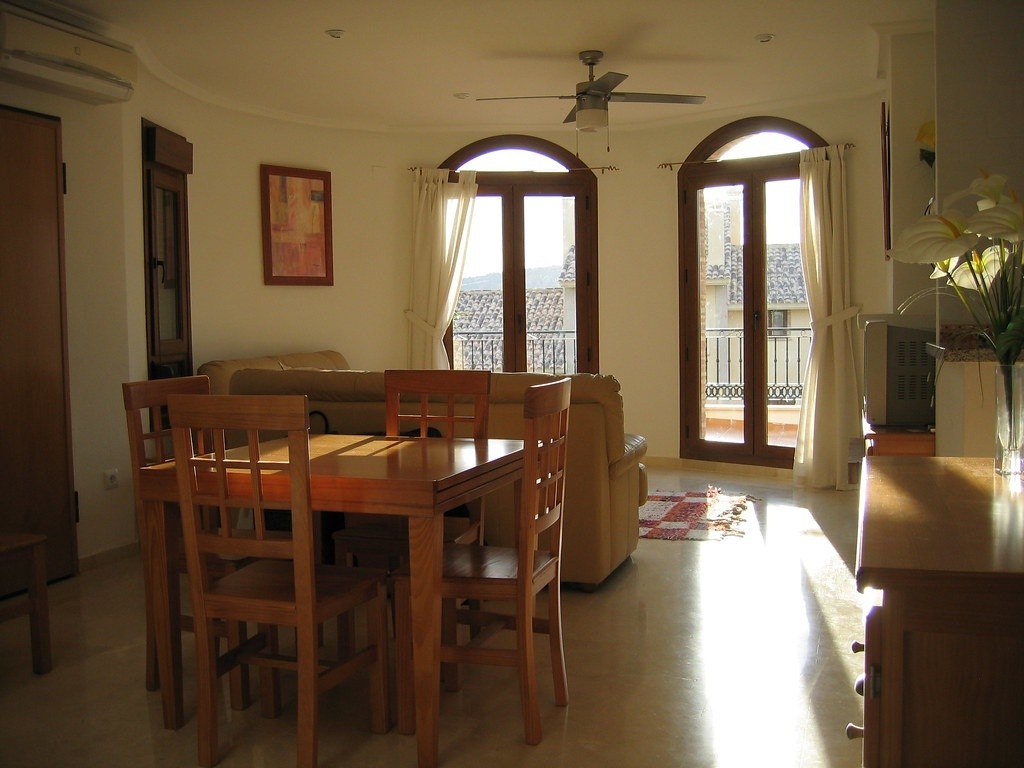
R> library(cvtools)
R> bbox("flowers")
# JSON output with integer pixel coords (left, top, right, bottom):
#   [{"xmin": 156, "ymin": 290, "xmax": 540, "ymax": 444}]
[{"xmin": 888, "ymin": 174, "xmax": 1022, "ymax": 360}]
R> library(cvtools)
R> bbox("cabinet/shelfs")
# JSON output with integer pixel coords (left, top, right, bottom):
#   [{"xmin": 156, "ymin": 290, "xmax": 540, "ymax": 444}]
[
  {"xmin": 845, "ymin": 452, "xmax": 1024, "ymax": 768},
  {"xmin": 0, "ymin": 112, "xmax": 87, "ymax": 672}
]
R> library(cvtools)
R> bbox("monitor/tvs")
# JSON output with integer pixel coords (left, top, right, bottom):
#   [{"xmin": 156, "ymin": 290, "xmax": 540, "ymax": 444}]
[{"xmin": 862, "ymin": 319, "xmax": 989, "ymax": 426}]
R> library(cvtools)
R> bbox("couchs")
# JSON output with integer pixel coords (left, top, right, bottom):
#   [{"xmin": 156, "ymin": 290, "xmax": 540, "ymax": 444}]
[{"xmin": 195, "ymin": 351, "xmax": 649, "ymax": 589}]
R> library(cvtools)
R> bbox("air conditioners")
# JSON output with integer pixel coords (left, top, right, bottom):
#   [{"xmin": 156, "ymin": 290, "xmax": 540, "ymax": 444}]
[{"xmin": 0, "ymin": 0, "xmax": 139, "ymax": 107}]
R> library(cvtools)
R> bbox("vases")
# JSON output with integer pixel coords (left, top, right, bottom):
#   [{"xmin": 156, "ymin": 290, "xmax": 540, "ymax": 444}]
[{"xmin": 930, "ymin": 326, "xmax": 1024, "ymax": 458}]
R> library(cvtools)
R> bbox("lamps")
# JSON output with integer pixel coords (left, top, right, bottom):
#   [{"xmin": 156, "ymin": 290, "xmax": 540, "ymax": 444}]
[{"xmin": 576, "ymin": 109, "xmax": 611, "ymax": 156}]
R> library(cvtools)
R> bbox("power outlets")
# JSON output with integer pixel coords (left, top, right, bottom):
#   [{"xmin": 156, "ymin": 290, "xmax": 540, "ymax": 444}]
[{"xmin": 103, "ymin": 467, "xmax": 120, "ymax": 490}]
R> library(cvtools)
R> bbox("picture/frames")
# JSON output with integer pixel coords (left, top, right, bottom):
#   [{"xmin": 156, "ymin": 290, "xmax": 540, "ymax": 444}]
[
  {"xmin": 879, "ymin": 101, "xmax": 889, "ymax": 260},
  {"xmin": 260, "ymin": 164, "xmax": 335, "ymax": 287}
]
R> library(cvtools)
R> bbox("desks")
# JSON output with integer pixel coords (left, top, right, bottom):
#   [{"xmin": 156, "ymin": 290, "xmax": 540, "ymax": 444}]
[
  {"xmin": 861, "ymin": 410, "xmax": 938, "ymax": 455},
  {"xmin": 139, "ymin": 434, "xmax": 555, "ymax": 767}
]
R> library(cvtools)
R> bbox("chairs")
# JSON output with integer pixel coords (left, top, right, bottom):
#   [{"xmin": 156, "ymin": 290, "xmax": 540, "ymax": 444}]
[
  {"xmin": 121, "ymin": 368, "xmax": 572, "ymax": 767},
  {"xmin": 1, "ymin": 530, "xmax": 54, "ymax": 675}
]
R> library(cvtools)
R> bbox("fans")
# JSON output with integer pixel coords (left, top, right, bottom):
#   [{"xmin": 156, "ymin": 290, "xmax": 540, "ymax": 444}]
[{"xmin": 475, "ymin": 50, "xmax": 708, "ymax": 124}]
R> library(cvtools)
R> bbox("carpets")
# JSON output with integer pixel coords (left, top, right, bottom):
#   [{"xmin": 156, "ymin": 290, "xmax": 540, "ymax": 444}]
[{"xmin": 637, "ymin": 487, "xmax": 763, "ymax": 541}]
